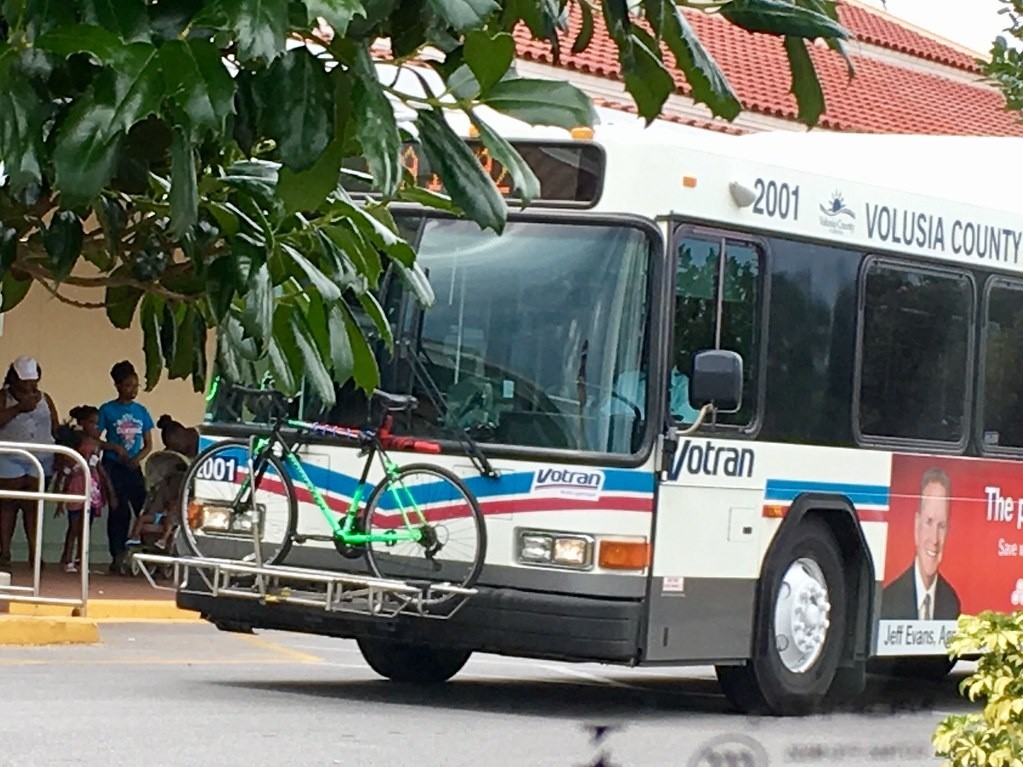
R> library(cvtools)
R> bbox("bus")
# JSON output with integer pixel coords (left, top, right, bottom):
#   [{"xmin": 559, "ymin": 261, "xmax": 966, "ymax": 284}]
[{"xmin": 170, "ymin": 94, "xmax": 1023, "ymax": 715}]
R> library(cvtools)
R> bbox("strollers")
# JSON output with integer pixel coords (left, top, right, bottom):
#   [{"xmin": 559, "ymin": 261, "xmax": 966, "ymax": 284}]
[{"xmin": 115, "ymin": 451, "xmax": 192, "ymax": 578}]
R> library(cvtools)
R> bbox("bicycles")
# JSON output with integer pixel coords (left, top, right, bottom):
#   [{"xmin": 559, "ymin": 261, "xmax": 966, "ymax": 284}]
[{"xmin": 174, "ymin": 378, "xmax": 488, "ymax": 612}]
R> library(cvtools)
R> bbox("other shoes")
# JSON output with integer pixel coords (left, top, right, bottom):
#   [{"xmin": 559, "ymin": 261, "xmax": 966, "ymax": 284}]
[
  {"xmin": 126, "ymin": 536, "xmax": 141, "ymax": 545},
  {"xmin": 108, "ymin": 556, "xmax": 123, "ymax": 571},
  {"xmin": 60, "ymin": 558, "xmax": 77, "ymax": 573},
  {"xmin": 0, "ymin": 553, "xmax": 12, "ymax": 572},
  {"xmin": 155, "ymin": 538, "xmax": 166, "ymax": 549}
]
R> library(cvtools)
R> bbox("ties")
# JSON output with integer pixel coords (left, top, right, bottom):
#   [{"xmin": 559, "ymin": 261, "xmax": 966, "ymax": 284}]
[{"xmin": 924, "ymin": 595, "xmax": 932, "ymax": 620}]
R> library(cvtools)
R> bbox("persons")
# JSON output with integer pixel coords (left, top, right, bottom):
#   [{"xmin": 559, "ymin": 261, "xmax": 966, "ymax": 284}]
[
  {"xmin": 0, "ymin": 355, "xmax": 59, "ymax": 571},
  {"xmin": 97, "ymin": 360, "xmax": 156, "ymax": 571},
  {"xmin": 599, "ymin": 319, "xmax": 700, "ymax": 429},
  {"xmin": 125, "ymin": 462, "xmax": 185, "ymax": 552},
  {"xmin": 146, "ymin": 409, "xmax": 200, "ymax": 461},
  {"xmin": 878, "ymin": 466, "xmax": 963, "ymax": 621},
  {"xmin": 56, "ymin": 405, "xmax": 117, "ymax": 570}
]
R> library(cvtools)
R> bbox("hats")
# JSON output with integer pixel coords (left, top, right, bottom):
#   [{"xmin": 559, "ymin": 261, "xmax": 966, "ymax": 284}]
[{"xmin": 13, "ymin": 355, "xmax": 39, "ymax": 381}]
